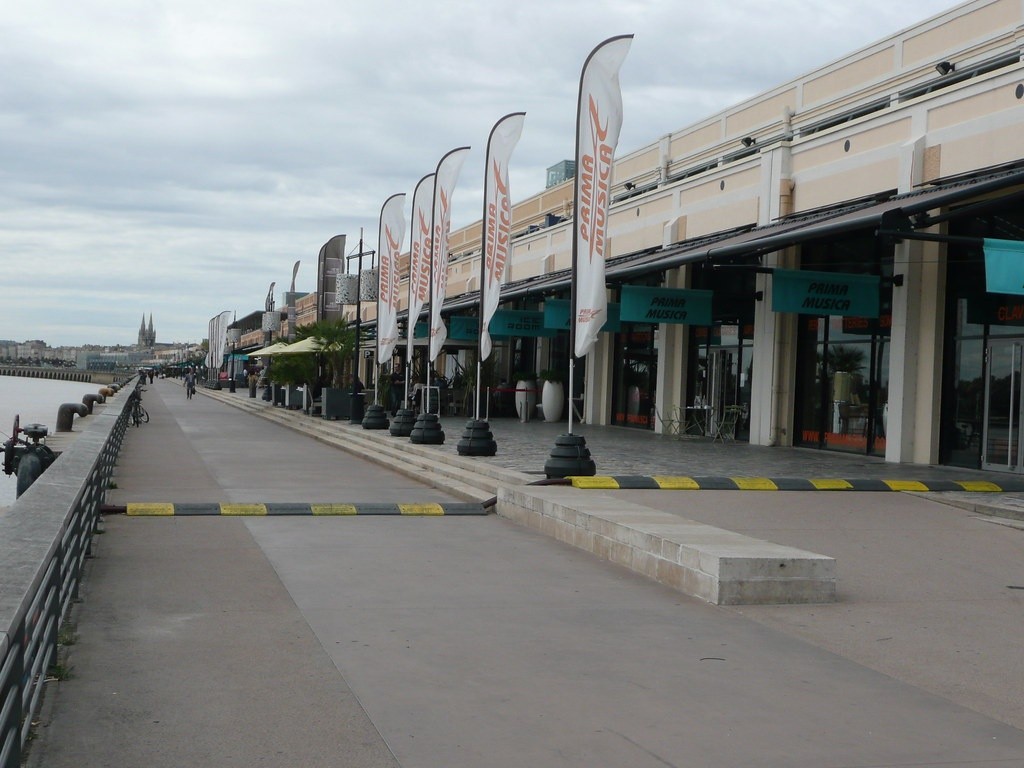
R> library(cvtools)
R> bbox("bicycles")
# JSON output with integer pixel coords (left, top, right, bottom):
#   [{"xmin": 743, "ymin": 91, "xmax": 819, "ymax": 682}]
[
  {"xmin": 183, "ymin": 382, "xmax": 195, "ymax": 399},
  {"xmin": 130, "ymin": 386, "xmax": 149, "ymax": 428}
]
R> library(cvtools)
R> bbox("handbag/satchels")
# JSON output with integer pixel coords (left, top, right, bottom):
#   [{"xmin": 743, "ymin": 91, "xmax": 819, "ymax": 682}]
[{"xmin": 192, "ymin": 386, "xmax": 195, "ymax": 394}]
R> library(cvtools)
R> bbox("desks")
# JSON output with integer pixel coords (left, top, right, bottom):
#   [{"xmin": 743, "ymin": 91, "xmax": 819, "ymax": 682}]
[{"xmin": 679, "ymin": 406, "xmax": 717, "ymax": 440}]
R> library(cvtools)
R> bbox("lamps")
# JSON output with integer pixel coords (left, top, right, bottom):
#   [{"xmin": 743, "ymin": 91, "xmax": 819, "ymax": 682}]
[
  {"xmin": 624, "ymin": 183, "xmax": 636, "ymax": 191},
  {"xmin": 741, "ymin": 137, "xmax": 757, "ymax": 148},
  {"xmin": 935, "ymin": 61, "xmax": 955, "ymax": 76}
]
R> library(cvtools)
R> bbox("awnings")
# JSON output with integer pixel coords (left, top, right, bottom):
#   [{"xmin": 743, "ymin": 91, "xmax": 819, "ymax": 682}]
[{"xmin": 344, "ymin": 160, "xmax": 1023, "ymax": 333}]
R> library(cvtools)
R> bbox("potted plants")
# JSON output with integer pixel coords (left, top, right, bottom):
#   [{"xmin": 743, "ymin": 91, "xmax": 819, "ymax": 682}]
[
  {"xmin": 512, "ymin": 369, "xmax": 536, "ymax": 418},
  {"xmin": 540, "ymin": 368, "xmax": 565, "ymax": 423},
  {"xmin": 625, "ymin": 365, "xmax": 647, "ymax": 425}
]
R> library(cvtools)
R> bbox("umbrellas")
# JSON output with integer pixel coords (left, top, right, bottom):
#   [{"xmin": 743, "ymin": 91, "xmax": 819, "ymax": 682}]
[
  {"xmin": 245, "ymin": 342, "xmax": 290, "ymax": 356},
  {"xmin": 274, "ymin": 336, "xmax": 356, "ymax": 376}
]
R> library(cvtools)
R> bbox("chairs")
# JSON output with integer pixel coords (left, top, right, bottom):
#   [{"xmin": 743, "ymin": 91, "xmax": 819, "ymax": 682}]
[
  {"xmin": 409, "ymin": 388, "xmax": 422, "ymax": 414},
  {"xmin": 489, "ymin": 391, "xmax": 516, "ymax": 418},
  {"xmin": 307, "ymin": 388, "xmax": 323, "ymax": 418},
  {"xmin": 656, "ymin": 404, "xmax": 742, "ymax": 445}
]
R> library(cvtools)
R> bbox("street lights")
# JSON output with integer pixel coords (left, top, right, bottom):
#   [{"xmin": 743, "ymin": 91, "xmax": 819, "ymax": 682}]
[{"xmin": 229, "ymin": 338, "xmax": 237, "ymax": 393}]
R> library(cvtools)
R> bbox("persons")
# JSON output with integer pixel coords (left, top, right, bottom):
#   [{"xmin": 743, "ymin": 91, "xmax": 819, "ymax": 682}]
[
  {"xmin": 353, "ymin": 372, "xmax": 517, "ymax": 405},
  {"xmin": 242, "ymin": 365, "xmax": 268, "ymax": 386},
  {"xmin": 182, "ymin": 370, "xmax": 195, "ymax": 399},
  {"xmin": 389, "ymin": 363, "xmax": 406, "ymax": 416},
  {"xmin": 140, "ymin": 367, "xmax": 178, "ymax": 386}
]
